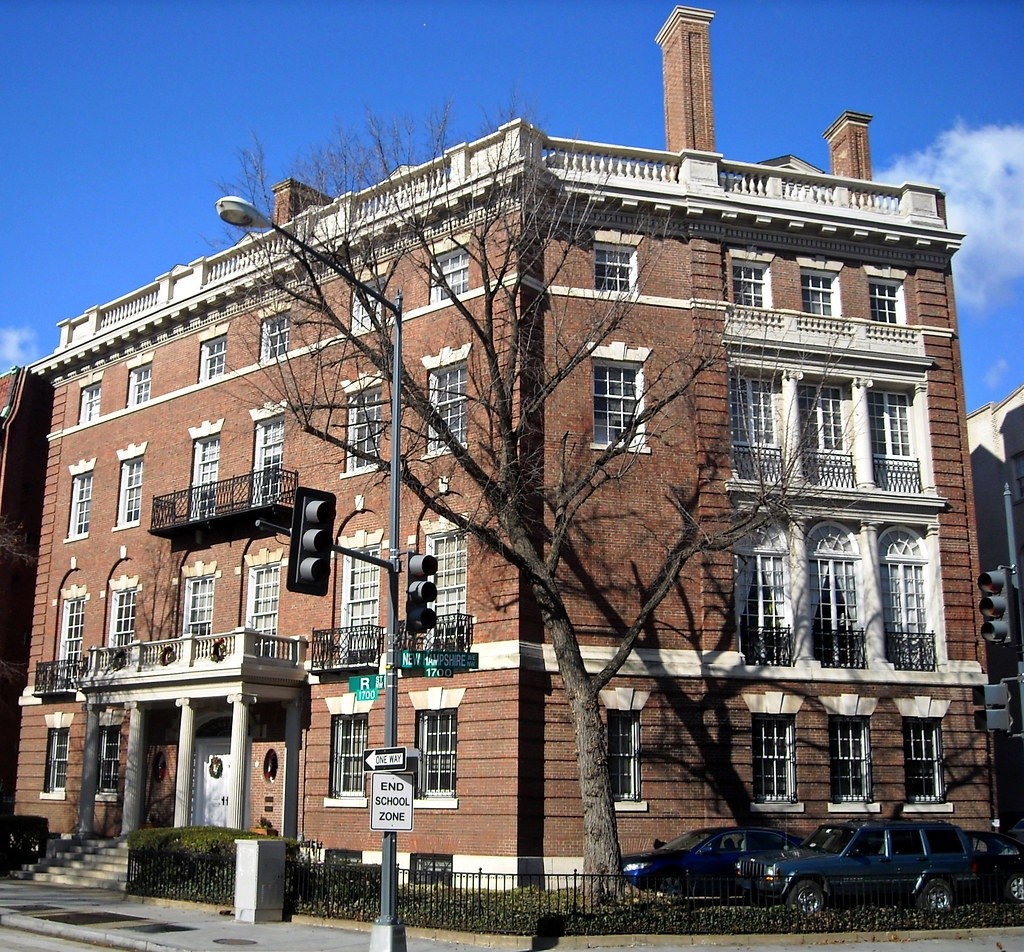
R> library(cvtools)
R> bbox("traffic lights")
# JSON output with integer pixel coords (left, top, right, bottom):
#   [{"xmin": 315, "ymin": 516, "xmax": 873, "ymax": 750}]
[
  {"xmin": 285, "ymin": 486, "xmax": 338, "ymax": 597},
  {"xmin": 977, "ymin": 568, "xmax": 1017, "ymax": 648},
  {"xmin": 404, "ymin": 551, "xmax": 437, "ymax": 634},
  {"xmin": 971, "ymin": 681, "xmax": 1012, "ymax": 732}
]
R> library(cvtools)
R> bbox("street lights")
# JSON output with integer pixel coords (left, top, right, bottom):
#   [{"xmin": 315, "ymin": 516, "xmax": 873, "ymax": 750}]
[{"xmin": 214, "ymin": 194, "xmax": 411, "ymax": 952}]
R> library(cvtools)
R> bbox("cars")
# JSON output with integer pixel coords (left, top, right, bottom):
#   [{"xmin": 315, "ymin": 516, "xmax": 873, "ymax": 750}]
[
  {"xmin": 1002, "ymin": 819, "xmax": 1024, "ymax": 844},
  {"xmin": 962, "ymin": 830, "xmax": 1024, "ymax": 904},
  {"xmin": 620, "ymin": 826, "xmax": 808, "ymax": 906}
]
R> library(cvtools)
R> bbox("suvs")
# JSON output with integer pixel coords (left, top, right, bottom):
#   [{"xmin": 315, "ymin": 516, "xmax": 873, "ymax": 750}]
[{"xmin": 733, "ymin": 815, "xmax": 984, "ymax": 913}]
